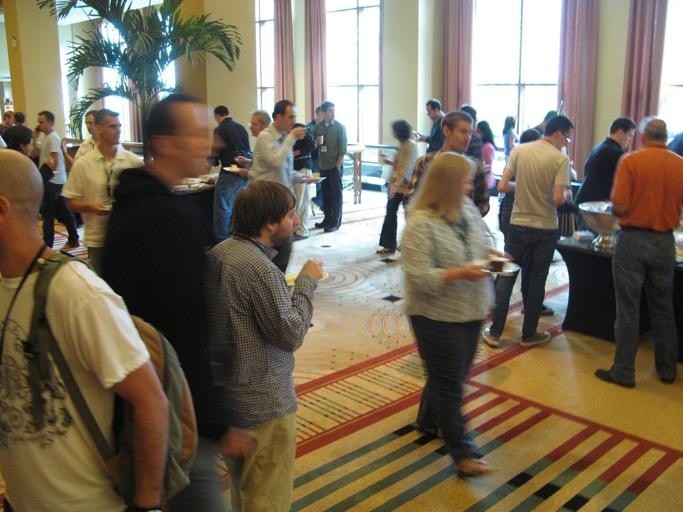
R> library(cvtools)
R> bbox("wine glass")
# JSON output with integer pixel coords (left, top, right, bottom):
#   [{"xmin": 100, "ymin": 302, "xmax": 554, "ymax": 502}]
[{"xmin": 578, "ymin": 200, "xmax": 621, "ymax": 248}]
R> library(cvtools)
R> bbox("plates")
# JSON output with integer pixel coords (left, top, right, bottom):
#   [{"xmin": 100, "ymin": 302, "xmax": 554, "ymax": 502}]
[
  {"xmin": 302, "ymin": 177, "xmax": 327, "ymax": 183},
  {"xmin": 99, "ymin": 205, "xmax": 112, "ymax": 211},
  {"xmin": 281, "ymin": 269, "xmax": 329, "ymax": 285},
  {"xmin": 462, "ymin": 260, "xmax": 519, "ymax": 274},
  {"xmin": 222, "ymin": 167, "xmax": 246, "ymax": 174}
]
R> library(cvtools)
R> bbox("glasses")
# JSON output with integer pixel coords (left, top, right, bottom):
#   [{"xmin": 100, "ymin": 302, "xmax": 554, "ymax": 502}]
[{"xmin": 561, "ymin": 132, "xmax": 571, "ymax": 144}]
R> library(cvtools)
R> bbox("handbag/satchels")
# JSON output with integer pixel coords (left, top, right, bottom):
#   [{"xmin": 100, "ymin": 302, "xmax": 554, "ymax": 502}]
[{"xmin": 116, "ymin": 315, "xmax": 197, "ymax": 510}]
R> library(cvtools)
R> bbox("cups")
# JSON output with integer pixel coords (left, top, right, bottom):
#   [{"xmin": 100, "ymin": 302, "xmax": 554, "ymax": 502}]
[
  {"xmin": 379, "ymin": 153, "xmax": 387, "ymax": 164},
  {"xmin": 318, "ymin": 135, "xmax": 325, "ymax": 145},
  {"xmin": 312, "ymin": 172, "xmax": 320, "ymax": 178},
  {"xmin": 297, "ymin": 127, "xmax": 306, "ymax": 140}
]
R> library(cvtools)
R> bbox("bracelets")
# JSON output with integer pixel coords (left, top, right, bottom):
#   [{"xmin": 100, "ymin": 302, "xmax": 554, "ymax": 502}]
[{"xmin": 125, "ymin": 501, "xmax": 161, "ymax": 511}]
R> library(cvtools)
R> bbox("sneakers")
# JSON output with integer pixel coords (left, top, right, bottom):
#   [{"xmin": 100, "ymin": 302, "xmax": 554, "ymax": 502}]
[
  {"xmin": 315, "ymin": 220, "xmax": 339, "ymax": 232},
  {"xmin": 482, "ymin": 328, "xmax": 500, "ymax": 347},
  {"xmin": 457, "ymin": 458, "xmax": 493, "ymax": 477},
  {"xmin": 595, "ymin": 369, "xmax": 634, "ymax": 388},
  {"xmin": 539, "ymin": 307, "xmax": 553, "ymax": 315},
  {"xmin": 292, "ymin": 234, "xmax": 308, "ymax": 241},
  {"xmin": 521, "ymin": 331, "xmax": 551, "ymax": 346},
  {"xmin": 376, "ymin": 248, "xmax": 396, "ymax": 253}
]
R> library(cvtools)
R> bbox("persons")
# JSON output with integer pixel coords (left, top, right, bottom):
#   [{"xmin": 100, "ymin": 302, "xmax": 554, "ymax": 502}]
[
  {"xmin": 368, "ymin": 97, "xmax": 683, "ymax": 319},
  {"xmin": 1, "ymin": 92, "xmax": 354, "ymax": 286},
  {"xmin": 103, "ymin": 94, "xmax": 259, "ymax": 511},
  {"xmin": 402, "ymin": 151, "xmax": 508, "ymax": 478},
  {"xmin": 1, "ymin": 149, "xmax": 174, "ymax": 511},
  {"xmin": 202, "ymin": 180, "xmax": 324, "ymax": 511},
  {"xmin": 477, "ymin": 118, "xmax": 571, "ymax": 347},
  {"xmin": 590, "ymin": 118, "xmax": 683, "ymax": 390}
]
